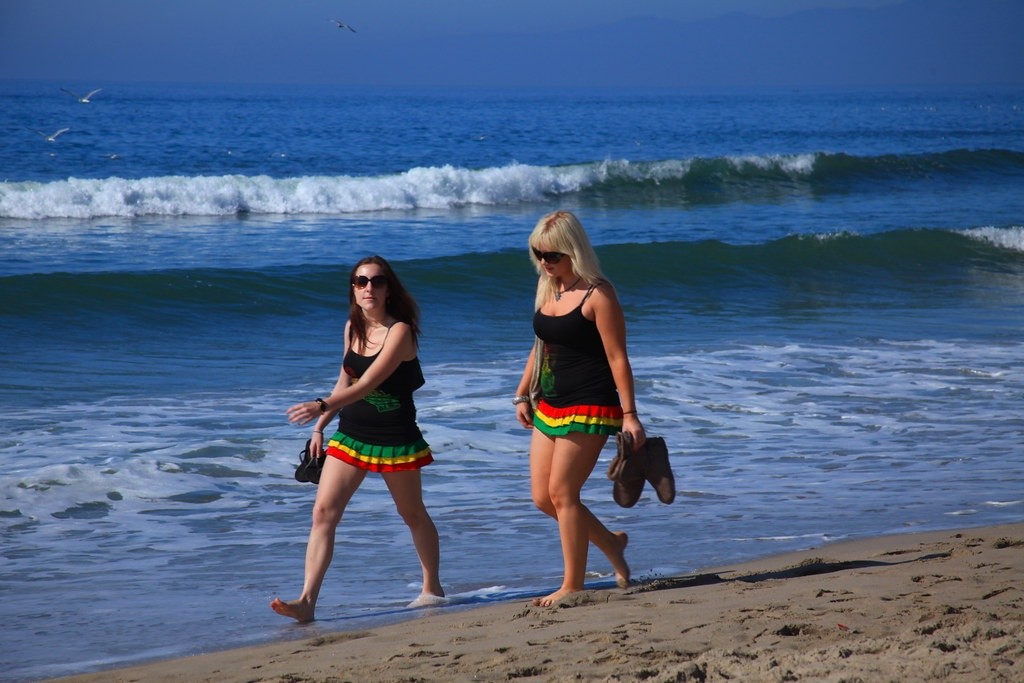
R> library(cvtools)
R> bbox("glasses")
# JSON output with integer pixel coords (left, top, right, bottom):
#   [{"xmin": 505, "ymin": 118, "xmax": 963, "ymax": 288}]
[
  {"xmin": 353, "ymin": 276, "xmax": 387, "ymax": 288},
  {"xmin": 532, "ymin": 248, "xmax": 566, "ymax": 264}
]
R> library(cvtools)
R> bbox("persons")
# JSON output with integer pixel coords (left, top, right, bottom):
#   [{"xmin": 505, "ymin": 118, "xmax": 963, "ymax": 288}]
[
  {"xmin": 516, "ymin": 210, "xmax": 646, "ymax": 608},
  {"xmin": 271, "ymin": 256, "xmax": 445, "ymax": 622}
]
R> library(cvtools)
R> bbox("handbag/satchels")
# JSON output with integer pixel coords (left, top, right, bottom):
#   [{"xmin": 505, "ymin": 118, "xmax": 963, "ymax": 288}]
[{"xmin": 529, "ymin": 335, "xmax": 543, "ymax": 410}]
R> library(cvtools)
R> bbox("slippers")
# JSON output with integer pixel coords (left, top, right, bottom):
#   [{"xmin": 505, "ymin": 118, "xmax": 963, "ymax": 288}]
[
  {"xmin": 295, "ymin": 439, "xmax": 321, "ymax": 482},
  {"xmin": 305, "ymin": 452, "xmax": 326, "ymax": 484}
]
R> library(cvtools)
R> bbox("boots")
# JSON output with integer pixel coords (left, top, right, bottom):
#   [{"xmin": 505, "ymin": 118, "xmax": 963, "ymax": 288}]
[
  {"xmin": 624, "ymin": 431, "xmax": 674, "ymax": 504},
  {"xmin": 608, "ymin": 432, "xmax": 645, "ymax": 507}
]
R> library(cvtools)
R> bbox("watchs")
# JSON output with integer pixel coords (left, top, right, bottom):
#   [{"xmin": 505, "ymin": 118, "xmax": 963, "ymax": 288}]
[{"xmin": 315, "ymin": 397, "xmax": 328, "ymax": 413}]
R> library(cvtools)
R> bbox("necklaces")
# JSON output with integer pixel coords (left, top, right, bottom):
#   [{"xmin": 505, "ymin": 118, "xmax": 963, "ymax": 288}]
[{"xmin": 553, "ymin": 278, "xmax": 580, "ymax": 300}]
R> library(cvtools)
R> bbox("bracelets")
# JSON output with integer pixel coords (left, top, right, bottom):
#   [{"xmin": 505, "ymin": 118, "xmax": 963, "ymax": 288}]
[
  {"xmin": 511, "ymin": 396, "xmax": 530, "ymax": 404},
  {"xmin": 622, "ymin": 410, "xmax": 637, "ymax": 414},
  {"xmin": 312, "ymin": 431, "xmax": 324, "ymax": 435}
]
